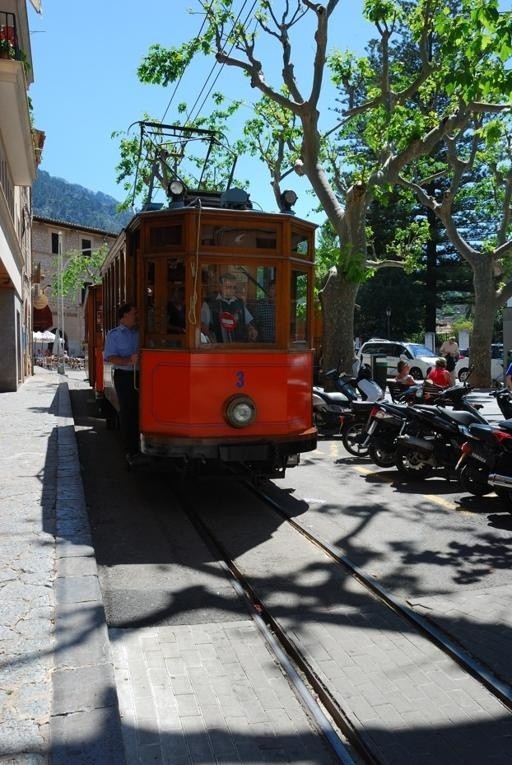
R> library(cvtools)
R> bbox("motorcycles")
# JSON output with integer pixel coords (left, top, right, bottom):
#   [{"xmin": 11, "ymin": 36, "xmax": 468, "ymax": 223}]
[{"xmin": 313, "ymin": 354, "xmax": 512, "ymax": 494}]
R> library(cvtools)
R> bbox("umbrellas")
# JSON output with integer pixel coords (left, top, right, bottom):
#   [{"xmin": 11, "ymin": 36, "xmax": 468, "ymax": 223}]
[{"xmin": 33, "ymin": 330, "xmax": 65, "ymax": 351}]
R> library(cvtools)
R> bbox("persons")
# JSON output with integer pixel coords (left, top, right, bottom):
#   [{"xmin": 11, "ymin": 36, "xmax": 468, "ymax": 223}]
[
  {"xmin": 201, "ymin": 273, "xmax": 258, "ymax": 343},
  {"xmin": 395, "ymin": 361, "xmax": 416, "ymax": 385},
  {"xmin": 427, "ymin": 357, "xmax": 452, "ymax": 389},
  {"xmin": 505, "ymin": 363, "xmax": 512, "ymax": 391},
  {"xmin": 440, "ymin": 336, "xmax": 460, "ymax": 386},
  {"xmin": 104, "ymin": 303, "xmax": 140, "ymax": 458},
  {"xmin": 253, "ymin": 280, "xmax": 276, "ymax": 343}
]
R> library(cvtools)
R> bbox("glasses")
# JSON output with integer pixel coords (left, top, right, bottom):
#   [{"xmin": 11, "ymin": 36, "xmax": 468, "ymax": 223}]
[{"xmin": 224, "ymin": 285, "xmax": 237, "ymax": 291}]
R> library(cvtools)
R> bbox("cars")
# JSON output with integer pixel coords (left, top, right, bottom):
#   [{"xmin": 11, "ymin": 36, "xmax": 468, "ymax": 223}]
[{"xmin": 355, "ymin": 339, "xmax": 503, "ymax": 382}]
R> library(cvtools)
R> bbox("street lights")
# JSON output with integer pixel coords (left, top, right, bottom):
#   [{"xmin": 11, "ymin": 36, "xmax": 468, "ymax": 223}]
[{"xmin": 386, "ymin": 306, "xmax": 391, "ymax": 336}]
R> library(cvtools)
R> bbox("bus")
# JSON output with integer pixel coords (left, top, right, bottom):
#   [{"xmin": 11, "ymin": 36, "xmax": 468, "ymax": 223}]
[{"xmin": 84, "ymin": 180, "xmax": 319, "ymax": 479}]
